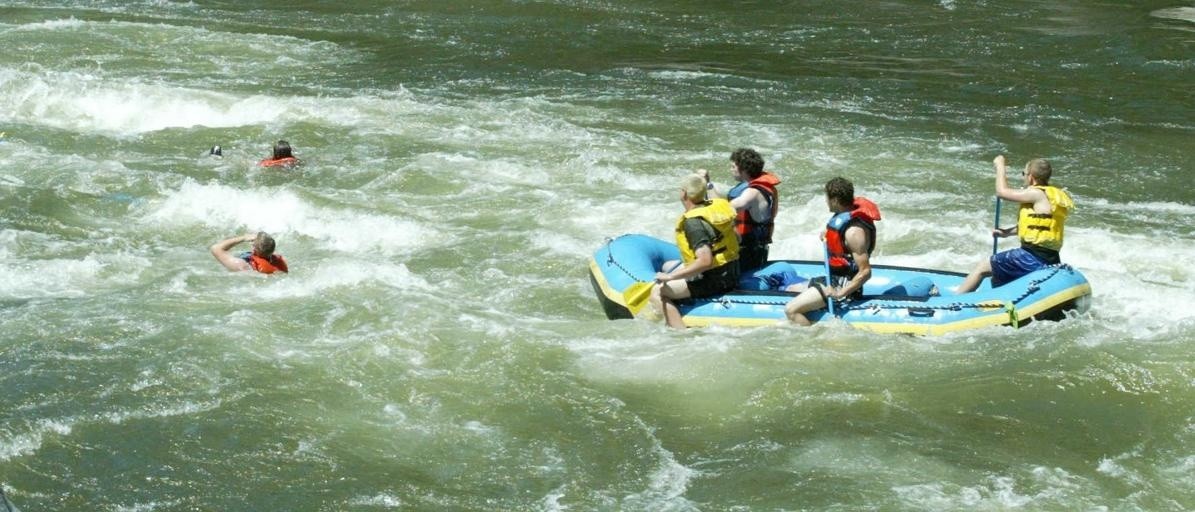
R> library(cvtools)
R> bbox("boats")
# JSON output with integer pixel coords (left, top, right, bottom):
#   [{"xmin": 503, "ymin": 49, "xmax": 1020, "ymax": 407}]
[{"xmin": 588, "ymin": 234, "xmax": 1090, "ymax": 338}]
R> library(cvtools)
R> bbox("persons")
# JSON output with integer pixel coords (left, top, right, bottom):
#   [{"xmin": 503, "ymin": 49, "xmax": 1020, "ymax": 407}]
[
  {"xmin": 697, "ymin": 149, "xmax": 781, "ymax": 270},
  {"xmin": 952, "ymin": 151, "xmax": 1078, "ymax": 296},
  {"xmin": 207, "ymin": 230, "xmax": 290, "ymax": 275},
  {"xmin": 783, "ymin": 176, "xmax": 882, "ymax": 328},
  {"xmin": 259, "ymin": 139, "xmax": 302, "ymax": 167},
  {"xmin": 650, "ymin": 173, "xmax": 741, "ymax": 332}
]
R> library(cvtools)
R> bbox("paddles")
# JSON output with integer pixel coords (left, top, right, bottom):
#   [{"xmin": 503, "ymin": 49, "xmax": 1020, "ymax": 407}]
[{"xmin": 623, "ymin": 260, "xmax": 684, "ymax": 315}]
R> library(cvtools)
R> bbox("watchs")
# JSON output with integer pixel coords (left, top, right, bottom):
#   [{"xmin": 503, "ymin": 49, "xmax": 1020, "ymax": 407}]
[{"xmin": 706, "ymin": 182, "xmax": 714, "ymax": 190}]
